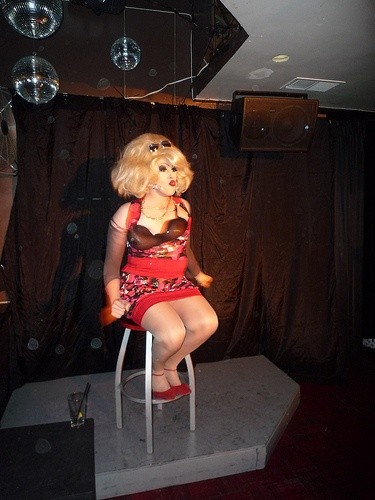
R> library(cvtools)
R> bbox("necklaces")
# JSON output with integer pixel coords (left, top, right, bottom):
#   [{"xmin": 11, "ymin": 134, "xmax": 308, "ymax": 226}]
[{"xmin": 140, "ymin": 195, "xmax": 179, "ymax": 219}]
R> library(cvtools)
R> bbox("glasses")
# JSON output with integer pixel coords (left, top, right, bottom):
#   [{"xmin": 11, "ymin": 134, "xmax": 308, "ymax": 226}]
[{"xmin": 148, "ymin": 140, "xmax": 171, "ymax": 152}]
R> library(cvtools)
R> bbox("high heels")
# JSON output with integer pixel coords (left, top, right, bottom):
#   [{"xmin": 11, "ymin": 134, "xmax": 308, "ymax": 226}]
[
  {"xmin": 164, "ymin": 368, "xmax": 191, "ymax": 395},
  {"xmin": 152, "ymin": 372, "xmax": 176, "ymax": 400}
]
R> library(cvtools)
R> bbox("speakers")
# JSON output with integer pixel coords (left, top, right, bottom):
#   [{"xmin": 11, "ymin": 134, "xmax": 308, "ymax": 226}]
[{"xmin": 230, "ymin": 97, "xmax": 319, "ymax": 151}]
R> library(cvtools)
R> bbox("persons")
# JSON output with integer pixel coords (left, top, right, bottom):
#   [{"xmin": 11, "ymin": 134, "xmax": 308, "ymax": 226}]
[{"xmin": 103, "ymin": 133, "xmax": 219, "ymax": 400}]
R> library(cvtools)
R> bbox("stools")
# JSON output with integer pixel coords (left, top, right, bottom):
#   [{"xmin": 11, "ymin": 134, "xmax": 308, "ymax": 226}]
[{"xmin": 114, "ymin": 319, "xmax": 196, "ymax": 455}]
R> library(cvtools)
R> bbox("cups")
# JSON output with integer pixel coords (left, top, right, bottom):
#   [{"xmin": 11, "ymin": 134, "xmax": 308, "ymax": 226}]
[{"xmin": 67, "ymin": 392, "xmax": 87, "ymax": 425}]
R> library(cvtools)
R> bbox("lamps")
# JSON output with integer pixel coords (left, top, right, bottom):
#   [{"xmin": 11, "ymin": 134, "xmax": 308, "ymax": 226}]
[
  {"xmin": 0, "ymin": 0, "xmax": 64, "ymax": 40},
  {"xmin": 108, "ymin": 33, "xmax": 142, "ymax": 72},
  {"xmin": 11, "ymin": 39, "xmax": 60, "ymax": 105}
]
